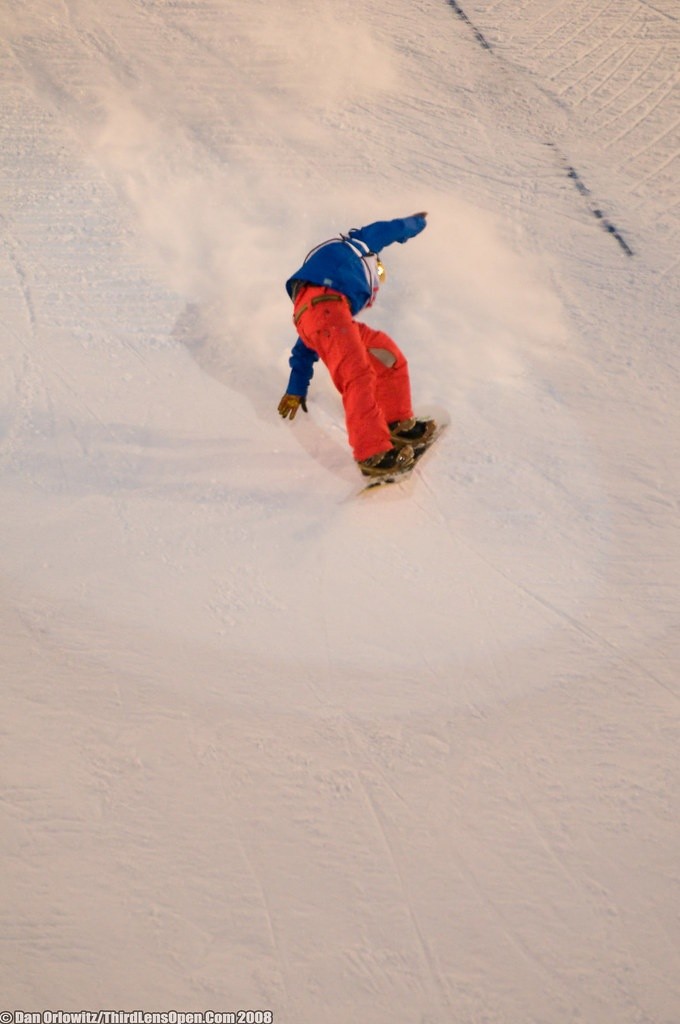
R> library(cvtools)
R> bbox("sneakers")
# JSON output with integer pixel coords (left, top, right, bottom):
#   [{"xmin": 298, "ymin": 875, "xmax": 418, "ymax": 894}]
[
  {"xmin": 359, "ymin": 445, "xmax": 414, "ymax": 487},
  {"xmin": 388, "ymin": 416, "xmax": 438, "ymax": 448}
]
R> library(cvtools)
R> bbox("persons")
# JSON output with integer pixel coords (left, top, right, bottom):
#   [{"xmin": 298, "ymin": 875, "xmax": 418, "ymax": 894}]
[{"xmin": 277, "ymin": 211, "xmax": 435, "ymax": 476}]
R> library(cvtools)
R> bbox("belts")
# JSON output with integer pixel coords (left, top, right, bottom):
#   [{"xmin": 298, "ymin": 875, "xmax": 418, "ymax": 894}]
[{"xmin": 294, "ymin": 295, "xmax": 343, "ymax": 323}]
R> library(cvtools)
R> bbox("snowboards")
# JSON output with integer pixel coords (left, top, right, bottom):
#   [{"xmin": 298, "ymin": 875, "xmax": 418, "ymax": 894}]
[{"xmin": 354, "ymin": 407, "xmax": 452, "ymax": 497}]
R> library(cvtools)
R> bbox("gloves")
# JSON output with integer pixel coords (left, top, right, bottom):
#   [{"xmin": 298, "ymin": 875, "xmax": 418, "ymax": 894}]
[{"xmin": 277, "ymin": 393, "xmax": 308, "ymax": 421}]
